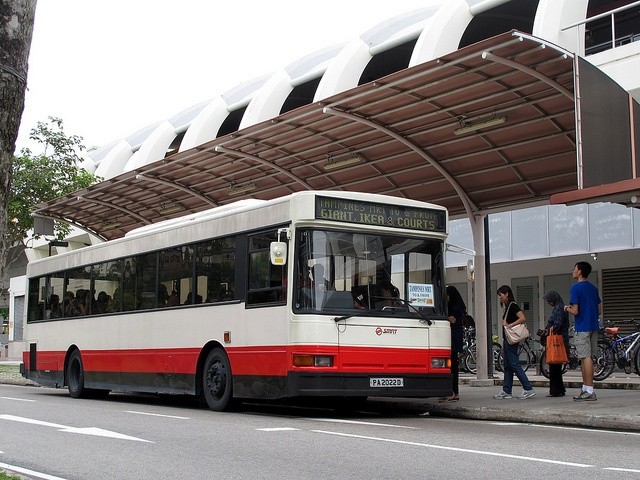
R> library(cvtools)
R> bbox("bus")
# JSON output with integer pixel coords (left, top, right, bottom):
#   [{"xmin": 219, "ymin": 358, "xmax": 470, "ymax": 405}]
[{"xmin": 20, "ymin": 191, "xmax": 477, "ymax": 413}]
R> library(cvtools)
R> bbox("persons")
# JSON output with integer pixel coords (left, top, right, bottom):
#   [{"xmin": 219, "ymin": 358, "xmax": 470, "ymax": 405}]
[
  {"xmin": 283, "ymin": 265, "xmax": 314, "ymax": 289},
  {"xmin": 49, "ymin": 286, "xmax": 122, "ymax": 319},
  {"xmin": 540, "ymin": 290, "xmax": 570, "ymax": 397},
  {"xmin": 159, "ymin": 282, "xmax": 174, "ymax": 308},
  {"xmin": 493, "ymin": 285, "xmax": 536, "ymax": 401},
  {"xmin": 564, "ymin": 261, "xmax": 602, "ymax": 401},
  {"xmin": 304, "ymin": 263, "xmax": 336, "ymax": 290},
  {"xmin": 439, "ymin": 286, "xmax": 466, "ymax": 402},
  {"xmin": 373, "ymin": 282, "xmax": 404, "ymax": 310}
]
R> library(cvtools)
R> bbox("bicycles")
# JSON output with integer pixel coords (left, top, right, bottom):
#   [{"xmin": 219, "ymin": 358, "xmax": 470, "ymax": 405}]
[{"xmin": 459, "ymin": 318, "xmax": 639, "ymax": 382}]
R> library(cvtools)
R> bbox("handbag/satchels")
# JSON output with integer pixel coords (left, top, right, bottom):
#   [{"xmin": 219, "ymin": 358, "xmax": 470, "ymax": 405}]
[
  {"xmin": 504, "ymin": 323, "xmax": 529, "ymax": 345},
  {"xmin": 546, "ymin": 326, "xmax": 568, "ymax": 364}
]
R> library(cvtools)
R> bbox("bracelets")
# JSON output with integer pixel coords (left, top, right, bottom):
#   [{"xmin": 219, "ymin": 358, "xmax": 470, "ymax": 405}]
[{"xmin": 564, "ymin": 305, "xmax": 570, "ymax": 311}]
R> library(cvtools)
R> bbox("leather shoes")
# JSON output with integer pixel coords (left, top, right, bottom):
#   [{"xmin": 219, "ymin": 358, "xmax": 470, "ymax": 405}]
[{"xmin": 546, "ymin": 394, "xmax": 563, "ymax": 397}]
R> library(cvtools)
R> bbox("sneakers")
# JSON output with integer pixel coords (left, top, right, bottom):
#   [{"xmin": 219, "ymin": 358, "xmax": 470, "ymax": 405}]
[
  {"xmin": 573, "ymin": 391, "xmax": 595, "ymax": 400},
  {"xmin": 516, "ymin": 389, "xmax": 536, "ymax": 399},
  {"xmin": 581, "ymin": 388, "xmax": 597, "ymax": 400},
  {"xmin": 493, "ymin": 389, "xmax": 512, "ymax": 399},
  {"xmin": 448, "ymin": 397, "xmax": 459, "ymax": 401}
]
